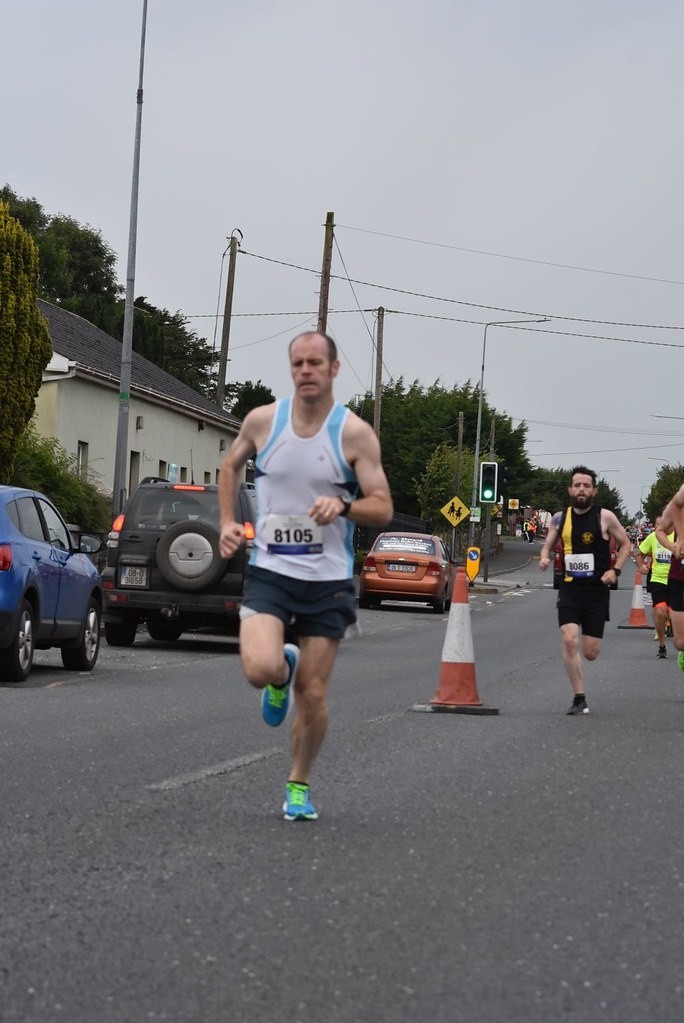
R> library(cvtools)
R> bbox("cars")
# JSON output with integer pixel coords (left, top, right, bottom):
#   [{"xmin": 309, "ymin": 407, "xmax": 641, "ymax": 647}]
[
  {"xmin": 0, "ymin": 484, "xmax": 105, "ymax": 683},
  {"xmin": 551, "ymin": 532, "xmax": 619, "ymax": 590},
  {"xmin": 358, "ymin": 531, "xmax": 454, "ymax": 614}
]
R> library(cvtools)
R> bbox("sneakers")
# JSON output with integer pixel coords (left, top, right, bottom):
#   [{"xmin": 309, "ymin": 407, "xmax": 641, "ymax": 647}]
[
  {"xmin": 564, "ymin": 694, "xmax": 588, "ymax": 715},
  {"xmin": 657, "ymin": 644, "xmax": 668, "ymax": 659},
  {"xmin": 281, "ymin": 779, "xmax": 320, "ymax": 822},
  {"xmin": 677, "ymin": 651, "xmax": 684, "ymax": 673},
  {"xmin": 258, "ymin": 642, "xmax": 300, "ymax": 727}
]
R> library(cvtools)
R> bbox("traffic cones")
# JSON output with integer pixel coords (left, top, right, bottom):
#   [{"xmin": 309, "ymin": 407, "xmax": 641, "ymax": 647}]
[
  {"xmin": 628, "ymin": 540, "xmax": 635, "ymax": 558},
  {"xmin": 412, "ymin": 566, "xmax": 502, "ymax": 715},
  {"xmin": 618, "ymin": 569, "xmax": 655, "ymax": 629}
]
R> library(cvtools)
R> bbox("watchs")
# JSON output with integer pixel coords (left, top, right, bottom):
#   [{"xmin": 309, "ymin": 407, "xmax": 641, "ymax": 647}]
[{"xmin": 337, "ymin": 493, "xmax": 352, "ymax": 518}]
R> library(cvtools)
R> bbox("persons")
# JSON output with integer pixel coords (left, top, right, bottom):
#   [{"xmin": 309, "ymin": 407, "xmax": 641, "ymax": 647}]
[
  {"xmin": 521, "ymin": 463, "xmax": 684, "ymax": 714},
  {"xmin": 217, "ymin": 331, "xmax": 393, "ymax": 820}
]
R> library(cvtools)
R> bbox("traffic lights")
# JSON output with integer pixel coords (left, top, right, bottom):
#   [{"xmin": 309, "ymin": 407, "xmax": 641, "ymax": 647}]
[{"xmin": 478, "ymin": 462, "xmax": 498, "ymax": 503}]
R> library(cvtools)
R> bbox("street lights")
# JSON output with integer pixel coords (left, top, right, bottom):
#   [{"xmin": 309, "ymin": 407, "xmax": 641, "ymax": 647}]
[{"xmin": 468, "ymin": 318, "xmax": 553, "ymax": 548}]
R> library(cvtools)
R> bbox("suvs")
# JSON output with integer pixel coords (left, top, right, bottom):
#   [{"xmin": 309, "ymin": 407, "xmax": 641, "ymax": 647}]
[{"xmin": 99, "ymin": 476, "xmax": 258, "ymax": 647}]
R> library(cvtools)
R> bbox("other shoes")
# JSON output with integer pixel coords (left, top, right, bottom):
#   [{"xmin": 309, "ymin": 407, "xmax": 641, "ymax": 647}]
[{"xmin": 653, "ymin": 623, "xmax": 674, "ymax": 640}]
